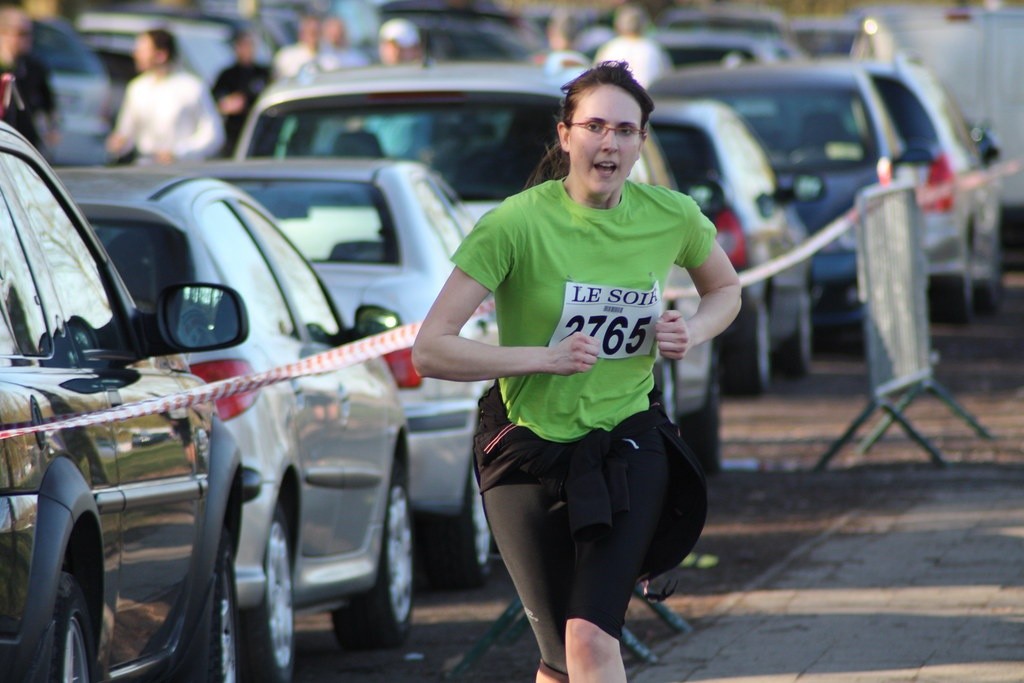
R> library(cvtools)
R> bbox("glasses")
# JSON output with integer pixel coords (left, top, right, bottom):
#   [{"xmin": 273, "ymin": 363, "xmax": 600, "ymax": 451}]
[{"xmin": 569, "ymin": 121, "xmax": 645, "ymax": 137}]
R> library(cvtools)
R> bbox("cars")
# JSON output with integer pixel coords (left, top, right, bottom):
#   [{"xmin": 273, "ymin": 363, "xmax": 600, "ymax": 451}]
[
  {"xmin": 135, "ymin": 152, "xmax": 500, "ymax": 594},
  {"xmin": 645, "ymin": 95, "xmax": 825, "ymax": 396},
  {"xmin": 378, "ymin": 0, "xmax": 1024, "ymax": 363},
  {"xmin": 52, "ymin": 162, "xmax": 419, "ymax": 683}
]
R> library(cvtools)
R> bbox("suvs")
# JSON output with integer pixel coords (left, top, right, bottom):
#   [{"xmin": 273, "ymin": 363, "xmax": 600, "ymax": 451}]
[
  {"xmin": 232, "ymin": 41, "xmax": 729, "ymax": 476},
  {"xmin": 0, "ymin": 120, "xmax": 249, "ymax": 683}
]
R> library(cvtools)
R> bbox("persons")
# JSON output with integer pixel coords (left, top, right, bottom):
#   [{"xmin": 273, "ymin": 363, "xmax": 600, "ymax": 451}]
[
  {"xmin": 2, "ymin": 0, "xmax": 676, "ymax": 164},
  {"xmin": 409, "ymin": 60, "xmax": 742, "ymax": 682}
]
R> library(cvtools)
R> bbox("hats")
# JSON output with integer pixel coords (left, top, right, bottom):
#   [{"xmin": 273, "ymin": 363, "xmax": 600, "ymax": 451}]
[{"xmin": 381, "ymin": 18, "xmax": 422, "ymax": 49}]
